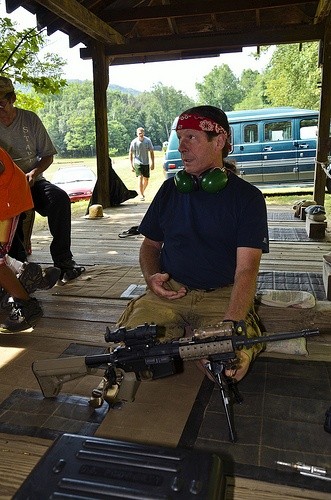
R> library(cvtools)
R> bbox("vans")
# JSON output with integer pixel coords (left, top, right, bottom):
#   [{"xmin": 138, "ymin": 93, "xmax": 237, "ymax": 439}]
[{"xmin": 164, "ymin": 106, "xmax": 326, "ymax": 192}]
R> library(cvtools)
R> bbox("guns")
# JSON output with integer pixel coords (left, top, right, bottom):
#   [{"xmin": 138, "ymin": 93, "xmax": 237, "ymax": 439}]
[{"xmin": 33, "ymin": 321, "xmax": 331, "ymax": 439}]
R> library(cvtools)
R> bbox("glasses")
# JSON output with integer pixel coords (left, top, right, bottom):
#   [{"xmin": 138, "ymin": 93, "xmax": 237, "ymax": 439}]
[{"xmin": 0, "ymin": 101, "xmax": 8, "ymax": 108}]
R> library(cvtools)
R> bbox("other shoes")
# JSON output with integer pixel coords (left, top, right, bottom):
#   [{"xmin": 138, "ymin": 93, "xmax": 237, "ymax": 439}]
[
  {"xmin": 60, "ymin": 260, "xmax": 81, "ymax": 284},
  {"xmin": 0, "ymin": 298, "xmax": 44, "ymax": 334}
]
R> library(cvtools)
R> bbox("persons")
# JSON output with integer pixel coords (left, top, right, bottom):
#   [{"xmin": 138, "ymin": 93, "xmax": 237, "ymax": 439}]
[
  {"xmin": 108, "ymin": 105, "xmax": 270, "ymax": 384},
  {"xmin": 0, "ymin": 148, "xmax": 43, "ymax": 335},
  {"xmin": 0, "ymin": 76, "xmax": 86, "ymax": 287},
  {"xmin": 129, "ymin": 127, "xmax": 155, "ymax": 202}
]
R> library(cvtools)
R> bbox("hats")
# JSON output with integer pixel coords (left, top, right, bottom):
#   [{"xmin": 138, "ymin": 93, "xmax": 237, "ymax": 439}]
[{"xmin": 0, "ymin": 76, "xmax": 14, "ymax": 98}]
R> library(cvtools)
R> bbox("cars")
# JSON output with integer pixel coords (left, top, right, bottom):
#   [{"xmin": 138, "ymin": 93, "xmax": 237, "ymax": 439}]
[{"xmin": 53, "ymin": 166, "xmax": 97, "ymax": 203}]
